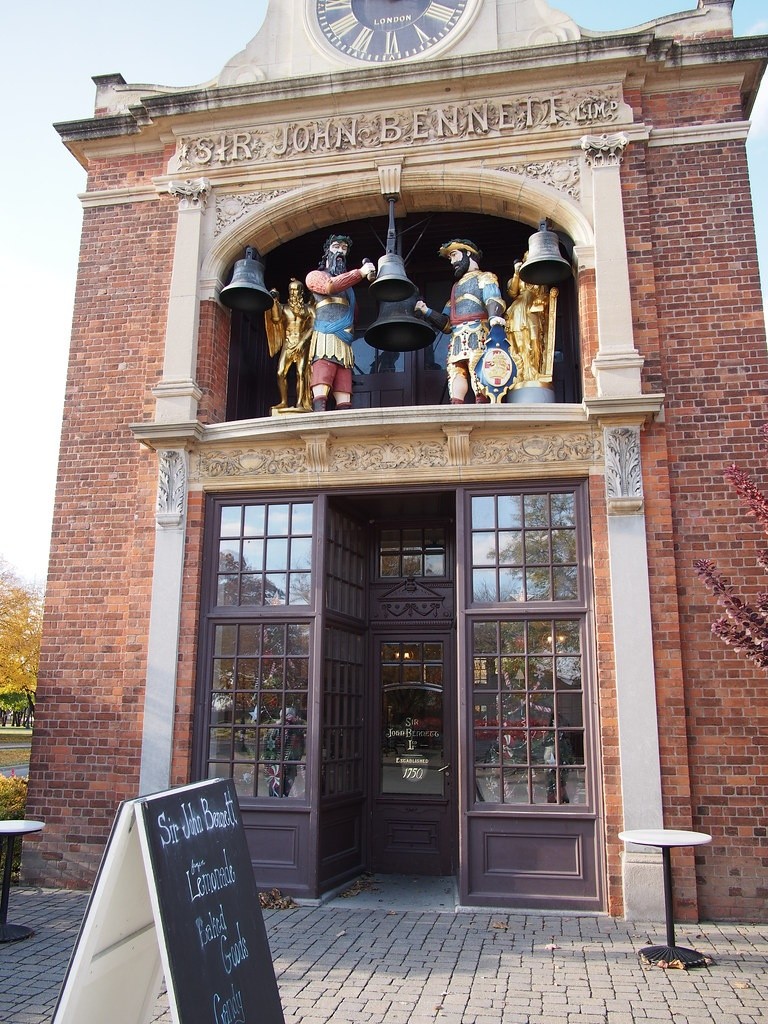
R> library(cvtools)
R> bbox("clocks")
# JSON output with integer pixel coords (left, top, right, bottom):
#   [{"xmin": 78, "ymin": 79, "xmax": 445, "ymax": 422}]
[{"xmin": 306, "ymin": 0, "xmax": 474, "ymax": 63}]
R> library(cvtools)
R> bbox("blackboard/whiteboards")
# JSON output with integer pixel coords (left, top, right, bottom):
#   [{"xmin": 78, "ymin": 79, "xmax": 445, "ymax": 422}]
[{"xmin": 51, "ymin": 777, "xmax": 295, "ymax": 1023}]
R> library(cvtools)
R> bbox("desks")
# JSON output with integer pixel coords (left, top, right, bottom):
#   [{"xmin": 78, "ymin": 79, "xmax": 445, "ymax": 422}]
[
  {"xmin": 0, "ymin": 819, "xmax": 45, "ymax": 944},
  {"xmin": 618, "ymin": 828, "xmax": 713, "ymax": 970}
]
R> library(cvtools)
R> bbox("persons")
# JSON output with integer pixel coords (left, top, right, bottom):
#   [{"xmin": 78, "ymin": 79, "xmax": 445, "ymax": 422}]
[
  {"xmin": 265, "ymin": 277, "xmax": 315, "ymax": 410},
  {"xmin": 505, "ymin": 249, "xmax": 548, "ymax": 389},
  {"xmin": 415, "ymin": 238, "xmax": 506, "ymax": 406},
  {"xmin": 543, "ymin": 711, "xmax": 579, "ymax": 804},
  {"xmin": 305, "ymin": 232, "xmax": 377, "ymax": 412},
  {"xmin": 263, "ymin": 708, "xmax": 307, "ymax": 798}
]
